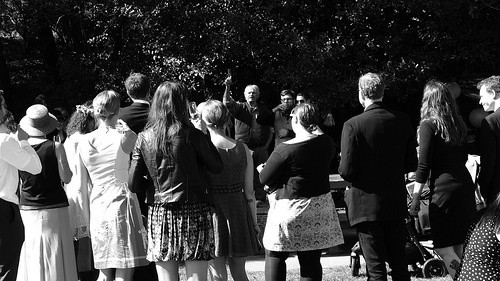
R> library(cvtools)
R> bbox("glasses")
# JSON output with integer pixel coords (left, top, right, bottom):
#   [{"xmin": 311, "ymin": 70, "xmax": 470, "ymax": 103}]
[{"xmin": 296, "ymin": 100, "xmax": 304, "ymax": 103}]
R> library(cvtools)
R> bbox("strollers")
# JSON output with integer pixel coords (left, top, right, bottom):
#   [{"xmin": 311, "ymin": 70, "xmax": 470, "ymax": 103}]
[{"xmin": 349, "ymin": 189, "xmax": 446, "ymax": 279}]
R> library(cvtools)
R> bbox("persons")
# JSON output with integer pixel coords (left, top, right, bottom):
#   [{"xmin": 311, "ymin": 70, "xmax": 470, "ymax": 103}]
[{"xmin": 1, "ymin": 69, "xmax": 500, "ymax": 281}]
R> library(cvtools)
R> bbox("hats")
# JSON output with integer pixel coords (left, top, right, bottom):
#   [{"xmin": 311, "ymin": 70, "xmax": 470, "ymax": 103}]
[{"xmin": 18, "ymin": 103, "xmax": 58, "ymax": 137}]
